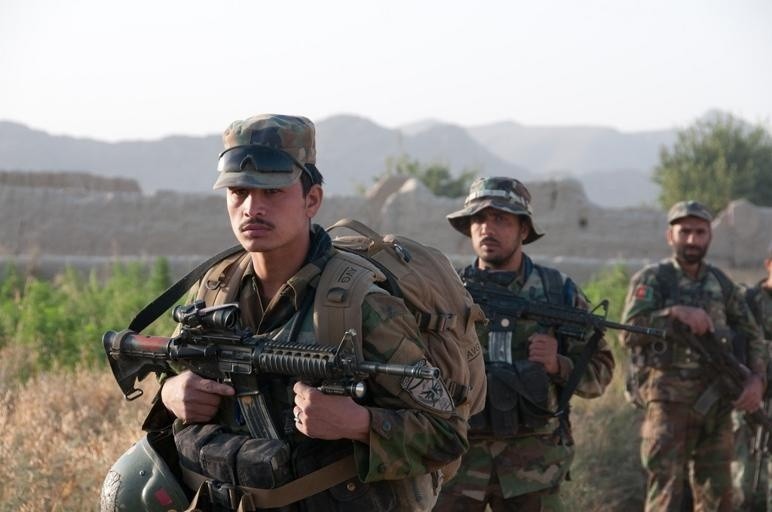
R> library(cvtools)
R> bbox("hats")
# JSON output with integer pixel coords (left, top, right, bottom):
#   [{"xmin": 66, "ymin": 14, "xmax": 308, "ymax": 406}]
[
  {"xmin": 447, "ymin": 175, "xmax": 544, "ymax": 245},
  {"xmin": 214, "ymin": 113, "xmax": 315, "ymax": 192},
  {"xmin": 667, "ymin": 201, "xmax": 716, "ymax": 223}
]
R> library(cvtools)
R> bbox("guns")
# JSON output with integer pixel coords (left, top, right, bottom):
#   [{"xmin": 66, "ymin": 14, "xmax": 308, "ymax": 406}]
[
  {"xmin": 102, "ymin": 300, "xmax": 440, "ymax": 439},
  {"xmin": 749, "ymin": 393, "xmax": 771, "ymax": 490},
  {"xmin": 677, "ymin": 321, "xmax": 772, "ymax": 429},
  {"xmin": 461, "ymin": 278, "xmax": 666, "ymax": 353}
]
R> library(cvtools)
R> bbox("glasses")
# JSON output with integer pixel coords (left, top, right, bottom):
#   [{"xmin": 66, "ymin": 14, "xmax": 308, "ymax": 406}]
[{"xmin": 214, "ymin": 143, "xmax": 317, "ymax": 188}]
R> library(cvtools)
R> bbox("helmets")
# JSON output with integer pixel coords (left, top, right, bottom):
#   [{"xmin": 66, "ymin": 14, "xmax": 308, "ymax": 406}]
[{"xmin": 98, "ymin": 431, "xmax": 194, "ymax": 512}]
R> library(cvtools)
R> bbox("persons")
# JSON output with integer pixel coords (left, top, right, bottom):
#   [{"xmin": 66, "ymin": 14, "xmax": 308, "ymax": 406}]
[
  {"xmin": 618, "ymin": 199, "xmax": 768, "ymax": 512},
  {"xmin": 729, "ymin": 247, "xmax": 772, "ymax": 512},
  {"xmin": 142, "ymin": 114, "xmax": 472, "ymax": 511},
  {"xmin": 433, "ymin": 173, "xmax": 614, "ymax": 511}
]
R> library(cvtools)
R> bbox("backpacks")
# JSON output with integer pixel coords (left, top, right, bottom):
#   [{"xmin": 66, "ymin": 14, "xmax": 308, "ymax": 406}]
[
  {"xmin": 622, "ymin": 340, "xmax": 651, "ymax": 411},
  {"xmin": 198, "ymin": 220, "xmax": 489, "ymax": 430}
]
